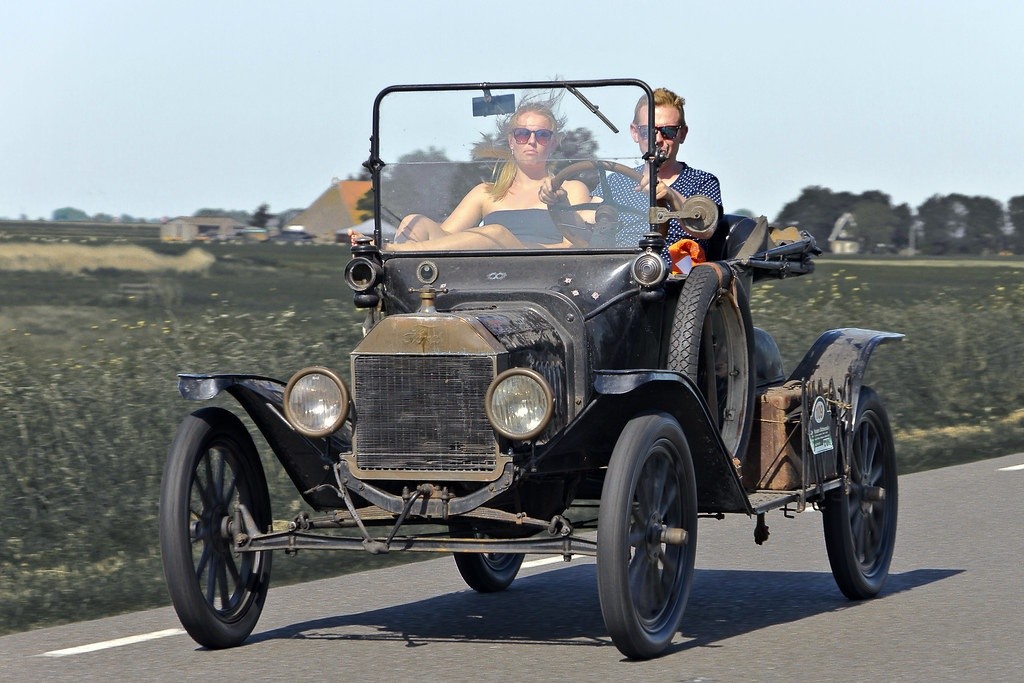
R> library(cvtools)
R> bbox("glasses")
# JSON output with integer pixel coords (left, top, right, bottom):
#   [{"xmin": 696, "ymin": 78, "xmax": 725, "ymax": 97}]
[
  {"xmin": 512, "ymin": 128, "xmax": 554, "ymax": 146},
  {"xmin": 638, "ymin": 122, "xmax": 685, "ymax": 140}
]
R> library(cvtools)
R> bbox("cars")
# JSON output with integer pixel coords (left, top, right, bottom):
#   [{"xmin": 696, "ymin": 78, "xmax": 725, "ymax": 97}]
[{"xmin": 158, "ymin": 78, "xmax": 909, "ymax": 661}]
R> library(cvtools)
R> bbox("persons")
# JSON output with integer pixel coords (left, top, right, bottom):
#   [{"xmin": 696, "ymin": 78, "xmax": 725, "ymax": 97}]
[
  {"xmin": 347, "ymin": 75, "xmax": 591, "ymax": 251},
  {"xmin": 538, "ymin": 87, "xmax": 724, "ymax": 275}
]
